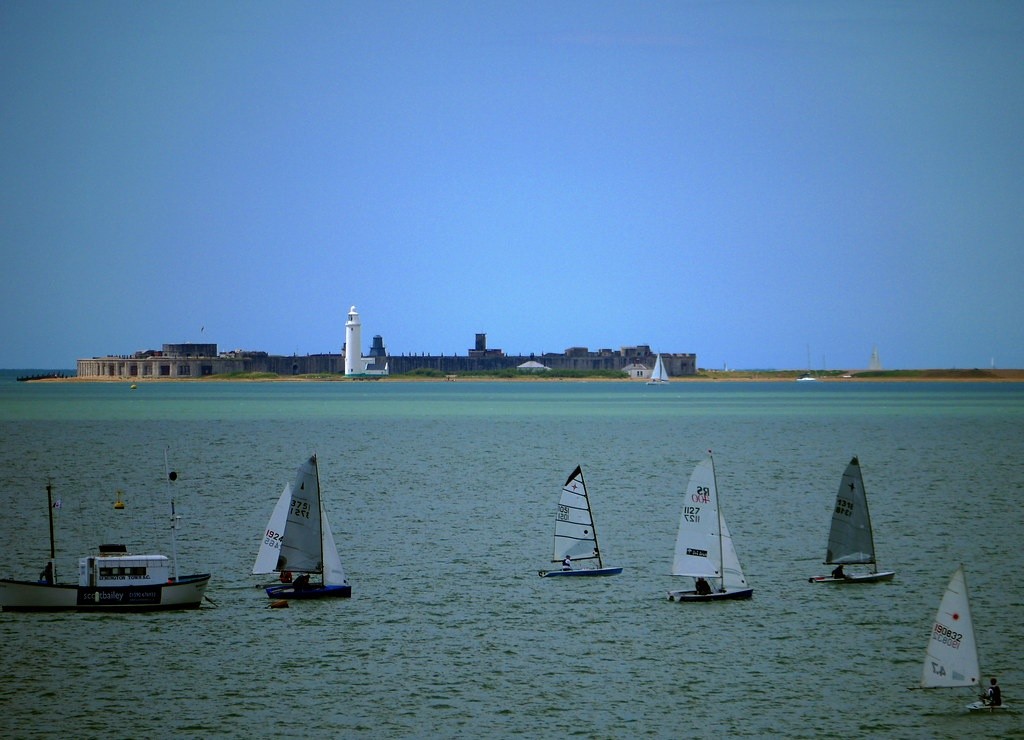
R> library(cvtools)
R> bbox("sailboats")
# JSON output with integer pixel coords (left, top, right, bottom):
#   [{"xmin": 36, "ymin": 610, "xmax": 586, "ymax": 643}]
[
  {"xmin": 248, "ymin": 447, "xmax": 353, "ymax": 602},
  {"xmin": 537, "ymin": 463, "xmax": 624, "ymax": 580},
  {"xmin": 645, "ymin": 348, "xmax": 672, "ymax": 386},
  {"xmin": 920, "ymin": 561, "xmax": 1012, "ymax": 716},
  {"xmin": 807, "ymin": 454, "xmax": 898, "ymax": 585},
  {"xmin": 663, "ymin": 447, "xmax": 756, "ymax": 605}
]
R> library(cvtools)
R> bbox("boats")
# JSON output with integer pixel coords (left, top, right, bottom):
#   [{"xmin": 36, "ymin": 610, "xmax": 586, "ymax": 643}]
[
  {"xmin": 796, "ymin": 371, "xmax": 817, "ymax": 382},
  {"xmin": 0, "ymin": 443, "xmax": 213, "ymax": 615}
]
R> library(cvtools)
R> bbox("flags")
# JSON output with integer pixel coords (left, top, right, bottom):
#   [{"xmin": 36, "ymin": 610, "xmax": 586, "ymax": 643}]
[
  {"xmin": 52, "ymin": 499, "xmax": 61, "ymax": 511},
  {"xmin": 201, "ymin": 326, "xmax": 204, "ymax": 332}
]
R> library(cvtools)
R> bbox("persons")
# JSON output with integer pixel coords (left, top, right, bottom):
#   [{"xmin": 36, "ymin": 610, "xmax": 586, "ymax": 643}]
[
  {"xmin": 562, "ymin": 555, "xmax": 573, "ymax": 571},
  {"xmin": 831, "ymin": 564, "xmax": 846, "ymax": 579},
  {"xmin": 39, "ymin": 562, "xmax": 57, "ymax": 584},
  {"xmin": 985, "ymin": 678, "xmax": 1001, "ymax": 706},
  {"xmin": 695, "ymin": 575, "xmax": 712, "ymax": 595},
  {"xmin": 280, "ymin": 571, "xmax": 310, "ymax": 593}
]
